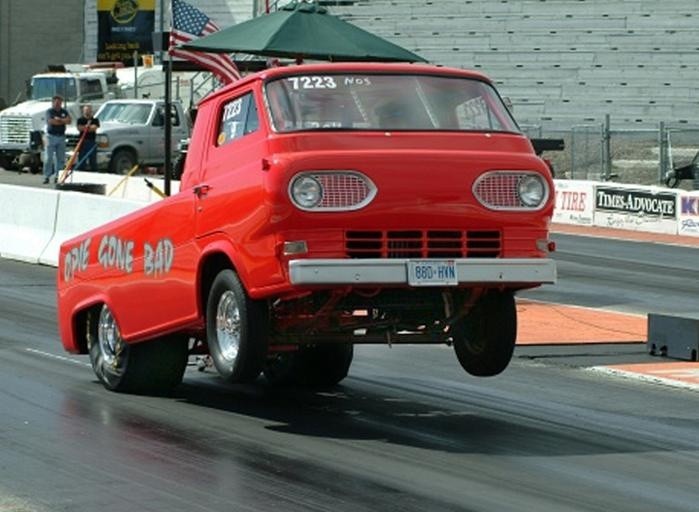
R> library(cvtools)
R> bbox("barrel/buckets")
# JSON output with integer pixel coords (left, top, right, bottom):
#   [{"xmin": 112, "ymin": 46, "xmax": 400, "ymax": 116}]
[{"xmin": 141, "ymin": 54, "xmax": 154, "ymax": 67}]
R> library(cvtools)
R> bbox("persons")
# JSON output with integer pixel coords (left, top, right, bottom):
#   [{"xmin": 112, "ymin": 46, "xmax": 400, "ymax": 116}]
[
  {"xmin": 42, "ymin": 96, "xmax": 72, "ymax": 187},
  {"xmin": 77, "ymin": 104, "xmax": 101, "ymax": 172}
]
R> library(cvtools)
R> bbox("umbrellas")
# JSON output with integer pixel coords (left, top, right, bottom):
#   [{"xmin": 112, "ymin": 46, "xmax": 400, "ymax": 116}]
[{"xmin": 174, "ymin": 1, "xmax": 427, "ymax": 68}]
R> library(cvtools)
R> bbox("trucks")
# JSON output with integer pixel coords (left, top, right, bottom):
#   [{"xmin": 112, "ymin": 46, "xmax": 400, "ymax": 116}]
[
  {"xmin": 57, "ymin": 63, "xmax": 556, "ymax": 394},
  {"xmin": 0, "ymin": 70, "xmax": 115, "ymax": 170}
]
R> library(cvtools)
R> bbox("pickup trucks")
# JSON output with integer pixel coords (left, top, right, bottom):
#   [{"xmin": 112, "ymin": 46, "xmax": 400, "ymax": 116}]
[{"xmin": 39, "ymin": 98, "xmax": 194, "ymax": 175}]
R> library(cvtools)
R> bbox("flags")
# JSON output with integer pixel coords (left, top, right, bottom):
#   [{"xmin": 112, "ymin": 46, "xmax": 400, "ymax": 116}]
[{"xmin": 169, "ymin": 0, "xmax": 241, "ymax": 86}]
